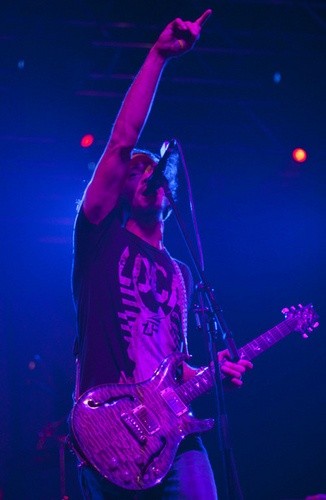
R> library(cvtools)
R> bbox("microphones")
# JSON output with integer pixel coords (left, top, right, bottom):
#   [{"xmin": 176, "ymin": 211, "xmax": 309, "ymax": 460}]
[{"xmin": 145, "ymin": 138, "xmax": 176, "ymax": 195}]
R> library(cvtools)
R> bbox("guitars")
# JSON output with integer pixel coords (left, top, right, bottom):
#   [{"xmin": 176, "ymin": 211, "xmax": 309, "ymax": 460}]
[{"xmin": 69, "ymin": 300, "xmax": 320, "ymax": 491}]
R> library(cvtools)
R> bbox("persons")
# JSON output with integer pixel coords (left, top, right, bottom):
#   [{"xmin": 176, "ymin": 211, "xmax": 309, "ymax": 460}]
[{"xmin": 66, "ymin": 8, "xmax": 253, "ymax": 500}]
